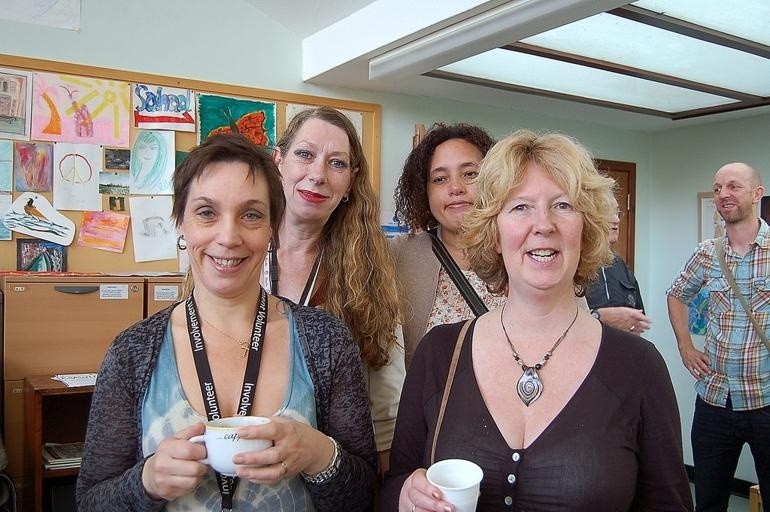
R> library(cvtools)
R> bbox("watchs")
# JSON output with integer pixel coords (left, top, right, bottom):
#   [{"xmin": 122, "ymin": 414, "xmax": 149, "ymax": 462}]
[{"xmin": 590, "ymin": 307, "xmax": 601, "ymax": 320}]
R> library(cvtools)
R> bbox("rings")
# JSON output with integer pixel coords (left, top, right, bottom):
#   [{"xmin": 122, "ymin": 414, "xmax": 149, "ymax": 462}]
[
  {"xmin": 278, "ymin": 460, "xmax": 289, "ymax": 476},
  {"xmin": 629, "ymin": 325, "xmax": 636, "ymax": 331},
  {"xmin": 411, "ymin": 504, "xmax": 418, "ymax": 512}
]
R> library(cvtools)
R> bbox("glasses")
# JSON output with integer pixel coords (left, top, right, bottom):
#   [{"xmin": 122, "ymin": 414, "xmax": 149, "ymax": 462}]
[{"xmin": 616, "ymin": 211, "xmax": 625, "ymax": 221}]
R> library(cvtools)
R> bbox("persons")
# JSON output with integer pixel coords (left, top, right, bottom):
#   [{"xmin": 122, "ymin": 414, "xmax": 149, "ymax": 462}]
[
  {"xmin": 664, "ymin": 162, "xmax": 770, "ymax": 510},
  {"xmin": 374, "ymin": 128, "xmax": 693, "ymax": 512},
  {"xmin": 582, "ymin": 188, "xmax": 653, "ymax": 341},
  {"xmin": 179, "ymin": 104, "xmax": 409, "ymax": 483},
  {"xmin": 73, "ymin": 131, "xmax": 385, "ymax": 511},
  {"xmin": 385, "ymin": 121, "xmax": 502, "ymax": 373}
]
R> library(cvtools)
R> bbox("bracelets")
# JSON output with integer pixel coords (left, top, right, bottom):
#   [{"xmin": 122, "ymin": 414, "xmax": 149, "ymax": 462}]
[{"xmin": 300, "ymin": 434, "xmax": 341, "ymax": 487}]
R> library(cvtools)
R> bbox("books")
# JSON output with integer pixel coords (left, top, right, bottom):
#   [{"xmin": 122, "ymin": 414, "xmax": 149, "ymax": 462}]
[{"xmin": 40, "ymin": 440, "xmax": 85, "ymax": 471}]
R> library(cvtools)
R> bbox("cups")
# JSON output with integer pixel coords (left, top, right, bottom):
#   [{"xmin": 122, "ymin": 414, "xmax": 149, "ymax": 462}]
[
  {"xmin": 189, "ymin": 416, "xmax": 274, "ymax": 477},
  {"xmin": 427, "ymin": 458, "xmax": 484, "ymax": 512}
]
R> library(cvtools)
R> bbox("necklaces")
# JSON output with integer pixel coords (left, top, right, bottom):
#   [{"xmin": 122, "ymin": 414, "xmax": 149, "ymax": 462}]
[
  {"xmin": 197, "ymin": 315, "xmax": 259, "ymax": 361},
  {"xmin": 488, "ymin": 298, "xmax": 582, "ymax": 407}
]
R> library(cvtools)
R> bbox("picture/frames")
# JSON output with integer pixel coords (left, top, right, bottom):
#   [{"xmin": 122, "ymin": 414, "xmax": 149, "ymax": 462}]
[{"xmin": 697, "ymin": 191, "xmax": 759, "ymax": 245}]
